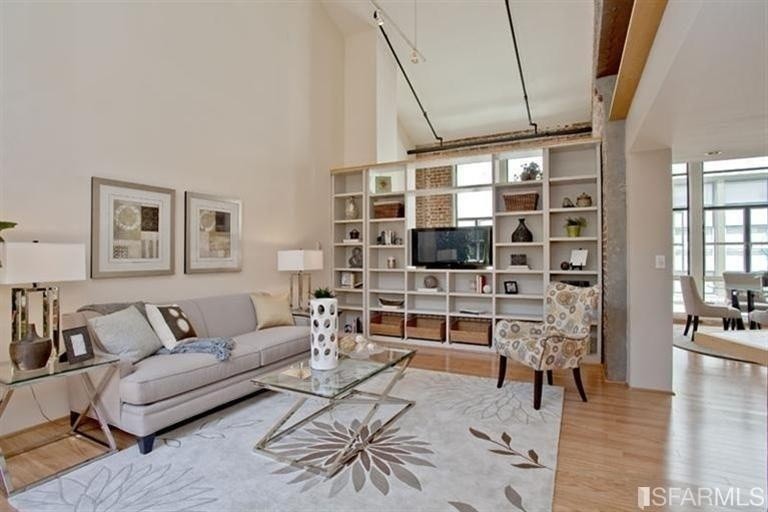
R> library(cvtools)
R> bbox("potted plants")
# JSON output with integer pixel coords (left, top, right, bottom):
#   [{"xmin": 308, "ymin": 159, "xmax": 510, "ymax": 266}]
[{"xmin": 561, "ymin": 215, "xmax": 588, "ymax": 238}]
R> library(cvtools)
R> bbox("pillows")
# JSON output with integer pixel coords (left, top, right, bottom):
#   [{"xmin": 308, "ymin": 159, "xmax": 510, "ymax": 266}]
[
  {"xmin": 251, "ymin": 294, "xmax": 297, "ymax": 332},
  {"xmin": 88, "ymin": 306, "xmax": 160, "ymax": 367},
  {"xmin": 147, "ymin": 302, "xmax": 195, "ymax": 347}
]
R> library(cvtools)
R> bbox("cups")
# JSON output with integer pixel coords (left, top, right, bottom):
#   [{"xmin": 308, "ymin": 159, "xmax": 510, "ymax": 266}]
[{"xmin": 387, "ymin": 257, "xmax": 395, "ymax": 268}]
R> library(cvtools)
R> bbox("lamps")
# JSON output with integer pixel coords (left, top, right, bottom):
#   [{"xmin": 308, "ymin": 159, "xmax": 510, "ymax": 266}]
[
  {"xmin": 277, "ymin": 251, "xmax": 324, "ymax": 313},
  {"xmin": 0, "ymin": 238, "xmax": 87, "ymax": 347}
]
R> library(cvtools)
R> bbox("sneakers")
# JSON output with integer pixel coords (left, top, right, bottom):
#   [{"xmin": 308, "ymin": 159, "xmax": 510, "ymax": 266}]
[{"xmin": 503, "ymin": 193, "xmax": 539, "ymax": 210}]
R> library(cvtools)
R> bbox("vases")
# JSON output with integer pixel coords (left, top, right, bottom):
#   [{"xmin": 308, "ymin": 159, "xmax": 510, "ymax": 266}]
[{"xmin": 511, "ymin": 216, "xmax": 533, "ymax": 241}]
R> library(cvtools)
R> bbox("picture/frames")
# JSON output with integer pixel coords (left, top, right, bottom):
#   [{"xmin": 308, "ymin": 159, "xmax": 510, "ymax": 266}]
[
  {"xmin": 62, "ymin": 327, "xmax": 94, "ymax": 364},
  {"xmin": 185, "ymin": 189, "xmax": 244, "ymax": 273},
  {"xmin": 90, "ymin": 176, "xmax": 176, "ymax": 279}
]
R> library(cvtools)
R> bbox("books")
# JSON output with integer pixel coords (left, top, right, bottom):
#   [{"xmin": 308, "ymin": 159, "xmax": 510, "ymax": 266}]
[
  {"xmin": 380, "ymin": 230, "xmax": 396, "ymax": 245},
  {"xmin": 475, "ymin": 274, "xmax": 486, "ymax": 294},
  {"xmin": 459, "ymin": 310, "xmax": 489, "ymax": 315}
]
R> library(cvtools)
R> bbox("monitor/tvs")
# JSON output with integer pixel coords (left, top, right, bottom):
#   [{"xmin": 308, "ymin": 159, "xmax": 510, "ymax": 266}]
[{"xmin": 411, "ymin": 225, "xmax": 493, "ymax": 269}]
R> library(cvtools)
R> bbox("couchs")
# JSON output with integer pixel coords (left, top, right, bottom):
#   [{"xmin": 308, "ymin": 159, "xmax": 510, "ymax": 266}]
[{"xmin": 65, "ymin": 294, "xmax": 314, "ymax": 453}]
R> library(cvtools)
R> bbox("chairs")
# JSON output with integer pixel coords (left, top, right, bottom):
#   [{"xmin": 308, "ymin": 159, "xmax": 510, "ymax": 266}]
[
  {"xmin": 679, "ymin": 269, "xmax": 768, "ymax": 342},
  {"xmin": 493, "ymin": 279, "xmax": 598, "ymax": 409}
]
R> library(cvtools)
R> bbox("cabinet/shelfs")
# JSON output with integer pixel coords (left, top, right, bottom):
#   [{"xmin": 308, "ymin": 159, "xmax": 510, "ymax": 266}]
[{"xmin": 330, "ymin": 140, "xmax": 604, "ymax": 365}]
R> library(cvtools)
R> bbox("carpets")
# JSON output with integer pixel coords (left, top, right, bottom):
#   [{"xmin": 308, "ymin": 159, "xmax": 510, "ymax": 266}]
[
  {"xmin": 7, "ymin": 351, "xmax": 564, "ymax": 511},
  {"xmin": 673, "ymin": 330, "xmax": 767, "ymax": 367}
]
